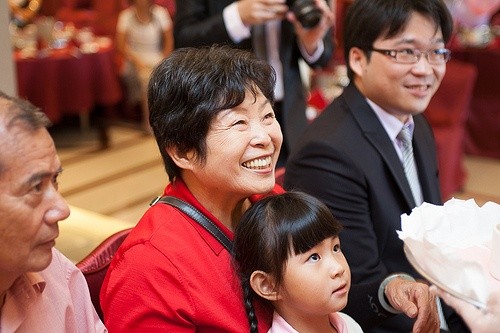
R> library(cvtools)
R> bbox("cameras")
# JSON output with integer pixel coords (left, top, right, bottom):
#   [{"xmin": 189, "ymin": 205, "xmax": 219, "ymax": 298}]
[{"xmin": 286, "ymin": 0, "xmax": 321, "ymax": 28}]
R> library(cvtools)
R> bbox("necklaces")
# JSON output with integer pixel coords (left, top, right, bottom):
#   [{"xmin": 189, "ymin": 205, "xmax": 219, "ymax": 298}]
[{"xmin": 134, "ymin": 5, "xmax": 153, "ymax": 25}]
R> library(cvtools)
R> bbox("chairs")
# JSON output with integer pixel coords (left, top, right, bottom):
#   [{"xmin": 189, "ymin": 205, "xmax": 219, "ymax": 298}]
[
  {"xmin": 421, "ymin": 61, "xmax": 476, "ymax": 200},
  {"xmin": 76, "ymin": 168, "xmax": 285, "ymax": 323}
]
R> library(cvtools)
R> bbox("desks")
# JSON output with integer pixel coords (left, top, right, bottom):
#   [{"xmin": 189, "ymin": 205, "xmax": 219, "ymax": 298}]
[{"xmin": 12, "ymin": 38, "xmax": 116, "ymax": 150}]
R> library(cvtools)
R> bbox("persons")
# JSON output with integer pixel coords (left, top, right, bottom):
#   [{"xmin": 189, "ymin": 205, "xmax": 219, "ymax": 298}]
[
  {"xmin": 283, "ymin": 0, "xmax": 469, "ymax": 333},
  {"xmin": 116, "ymin": 0, "xmax": 174, "ymax": 138},
  {"xmin": 100, "ymin": 45, "xmax": 291, "ymax": 333},
  {"xmin": 232, "ymin": 190, "xmax": 369, "ymax": 333},
  {"xmin": 0, "ymin": 93, "xmax": 109, "ymax": 333},
  {"xmin": 173, "ymin": 0, "xmax": 336, "ymax": 168},
  {"xmin": 1, "ymin": 0, "xmax": 41, "ymax": 99}
]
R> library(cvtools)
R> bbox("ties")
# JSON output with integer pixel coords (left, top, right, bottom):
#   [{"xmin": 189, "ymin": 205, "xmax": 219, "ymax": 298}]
[
  {"xmin": 253, "ymin": 22, "xmax": 268, "ymax": 63},
  {"xmin": 397, "ymin": 126, "xmax": 424, "ymax": 207}
]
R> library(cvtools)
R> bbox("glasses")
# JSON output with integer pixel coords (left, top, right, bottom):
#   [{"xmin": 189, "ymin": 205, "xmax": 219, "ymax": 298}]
[{"xmin": 359, "ymin": 47, "xmax": 451, "ymax": 65}]
